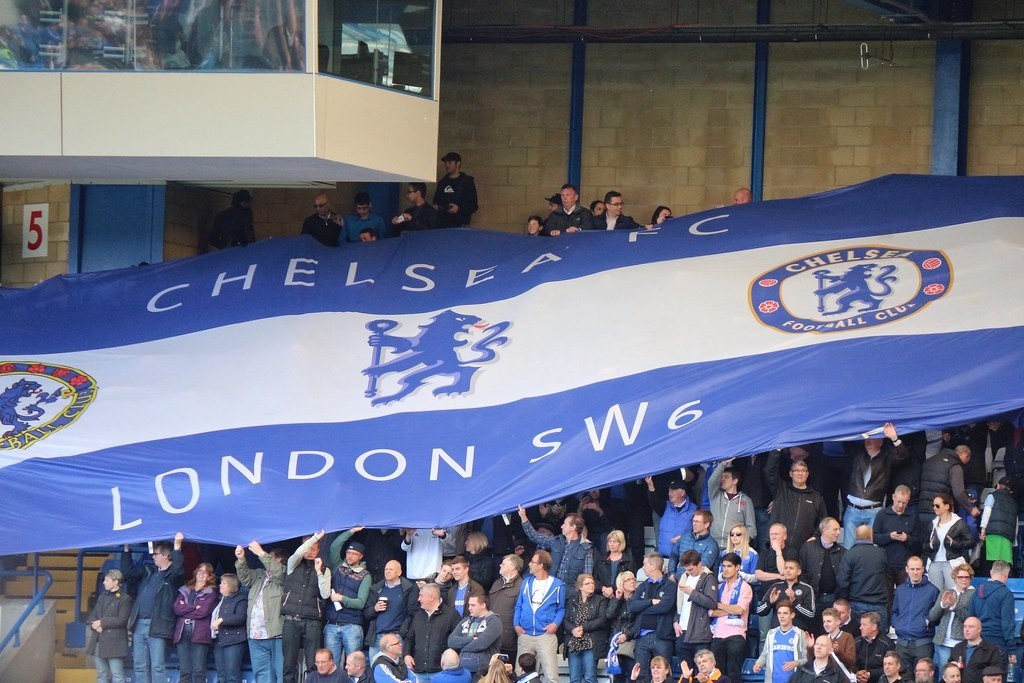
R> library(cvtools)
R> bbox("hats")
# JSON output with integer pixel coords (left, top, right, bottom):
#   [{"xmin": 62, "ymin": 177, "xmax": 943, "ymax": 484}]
[
  {"xmin": 536, "ymin": 522, "xmax": 556, "ymax": 536},
  {"xmin": 982, "ymin": 666, "xmax": 1005, "ymax": 677},
  {"xmin": 994, "ymin": 477, "xmax": 1013, "ymax": 489},
  {"xmin": 347, "ymin": 541, "xmax": 365, "ymax": 554},
  {"xmin": 966, "ymin": 488, "xmax": 979, "ymax": 500},
  {"xmin": 796, "ymin": 444, "xmax": 813, "ymax": 454},
  {"xmin": 667, "ymin": 480, "xmax": 688, "ymax": 490}
]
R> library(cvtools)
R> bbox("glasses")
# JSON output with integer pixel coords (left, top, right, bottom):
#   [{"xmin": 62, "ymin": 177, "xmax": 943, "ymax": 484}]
[
  {"xmin": 692, "ymin": 520, "xmax": 703, "ymax": 523},
  {"xmin": 315, "ymin": 662, "xmax": 328, "ymax": 665},
  {"xmin": 624, "ymin": 577, "xmax": 636, "ymax": 583},
  {"xmin": 914, "ymin": 669, "xmax": 926, "ymax": 673},
  {"xmin": 153, "ymin": 553, "xmax": 162, "ymax": 555},
  {"xmin": 793, "ymin": 469, "xmax": 808, "ymax": 474},
  {"xmin": 958, "ymin": 576, "xmax": 970, "ymax": 580},
  {"xmin": 932, "ymin": 504, "xmax": 939, "ymax": 508},
  {"xmin": 650, "ymin": 665, "xmax": 662, "ymax": 669},
  {"xmin": 730, "ymin": 531, "xmax": 742, "ymax": 536}
]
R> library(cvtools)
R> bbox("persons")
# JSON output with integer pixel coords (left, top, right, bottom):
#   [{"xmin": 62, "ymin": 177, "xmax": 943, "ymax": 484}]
[
  {"xmin": 301, "ymin": 193, "xmax": 340, "ymax": 247},
  {"xmin": 337, "ymin": 152, "xmax": 479, "ymax": 246},
  {"xmin": 82, "ymin": 405, "xmax": 1024, "ymax": 683},
  {"xmin": 208, "ymin": 189, "xmax": 256, "ymax": 251},
  {"xmin": 528, "ymin": 184, "xmax": 672, "ymax": 238},
  {"xmin": 734, "ymin": 188, "xmax": 752, "ymax": 205}
]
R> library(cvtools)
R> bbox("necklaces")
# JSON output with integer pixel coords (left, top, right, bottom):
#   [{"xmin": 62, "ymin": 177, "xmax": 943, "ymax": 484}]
[{"xmin": 829, "ymin": 630, "xmax": 840, "ymax": 639}]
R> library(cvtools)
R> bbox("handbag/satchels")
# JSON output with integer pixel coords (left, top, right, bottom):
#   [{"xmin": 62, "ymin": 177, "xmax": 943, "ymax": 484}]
[{"xmin": 616, "ymin": 638, "xmax": 637, "ymax": 660}]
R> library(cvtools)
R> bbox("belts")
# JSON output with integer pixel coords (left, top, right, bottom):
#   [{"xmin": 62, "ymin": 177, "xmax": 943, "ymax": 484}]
[
  {"xmin": 847, "ymin": 502, "xmax": 883, "ymax": 510},
  {"xmin": 329, "ymin": 621, "xmax": 351, "ymax": 626},
  {"xmin": 184, "ymin": 619, "xmax": 193, "ymax": 624},
  {"xmin": 286, "ymin": 615, "xmax": 303, "ymax": 621}
]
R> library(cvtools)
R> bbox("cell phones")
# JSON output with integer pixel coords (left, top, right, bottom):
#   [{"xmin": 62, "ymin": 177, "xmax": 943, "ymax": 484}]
[
  {"xmin": 897, "ymin": 531, "xmax": 902, "ymax": 534},
  {"xmin": 397, "ymin": 215, "xmax": 404, "ymax": 223},
  {"xmin": 498, "ymin": 654, "xmax": 509, "ymax": 661}
]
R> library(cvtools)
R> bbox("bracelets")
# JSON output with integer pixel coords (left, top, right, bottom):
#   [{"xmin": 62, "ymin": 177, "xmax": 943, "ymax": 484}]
[{"xmin": 892, "ymin": 438, "xmax": 899, "ymax": 443}]
